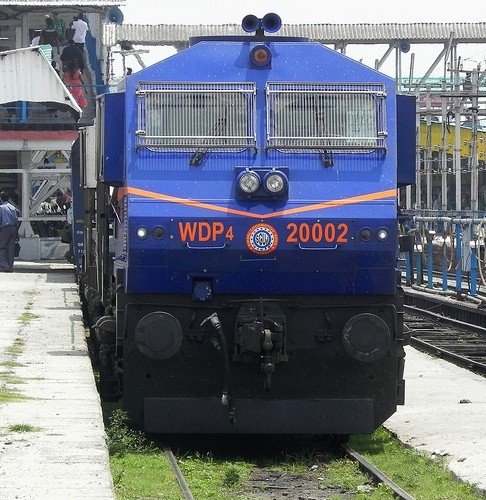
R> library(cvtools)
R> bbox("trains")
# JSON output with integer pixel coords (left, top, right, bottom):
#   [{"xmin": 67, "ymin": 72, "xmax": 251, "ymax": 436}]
[{"xmin": 65, "ymin": 13, "xmax": 418, "ymax": 442}]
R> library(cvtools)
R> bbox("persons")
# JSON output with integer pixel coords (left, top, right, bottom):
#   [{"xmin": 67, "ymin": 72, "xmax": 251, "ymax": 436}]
[
  {"xmin": 127, "ymin": 68, "xmax": 132, "ymax": 75},
  {"xmin": 0, "ymin": 191, "xmax": 21, "ymax": 272},
  {"xmin": 400, "ymin": 188, "xmax": 486, "ymax": 211},
  {"xmin": 29, "ymin": 10, "xmax": 92, "ymax": 109},
  {"xmin": 461, "ymin": 72, "xmax": 474, "ymax": 102},
  {"xmin": 34, "ymin": 186, "xmax": 72, "ymax": 238}
]
